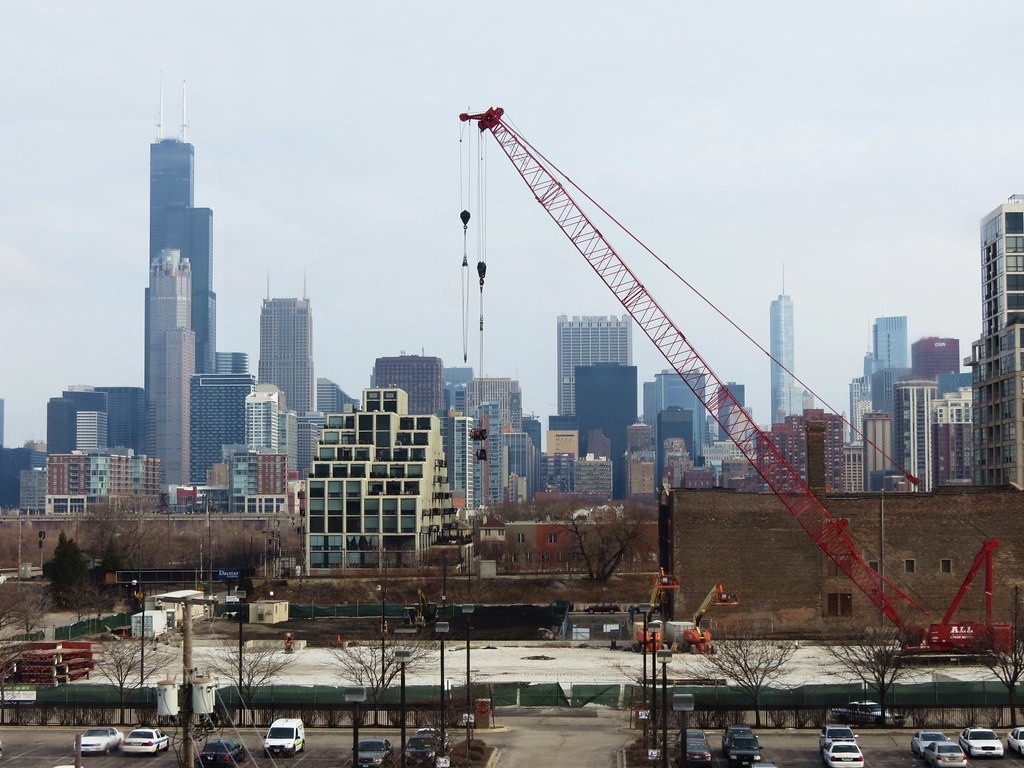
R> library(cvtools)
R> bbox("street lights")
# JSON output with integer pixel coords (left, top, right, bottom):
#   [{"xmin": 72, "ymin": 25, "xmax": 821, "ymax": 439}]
[
  {"xmin": 435, "ymin": 621, "xmax": 449, "ymax": 757},
  {"xmin": 462, "ymin": 603, "xmax": 474, "ymax": 750},
  {"xmin": 342, "ymin": 685, "xmax": 365, "ymax": 768},
  {"xmin": 395, "ymin": 650, "xmax": 411, "ymax": 768},
  {"xmin": 234, "ymin": 591, "xmax": 247, "ymax": 727},
  {"xmin": 647, "ymin": 622, "xmax": 660, "ymax": 750},
  {"xmin": 132, "ymin": 580, "xmax": 145, "ymax": 686},
  {"xmin": 657, "ymin": 650, "xmax": 673, "ymax": 768},
  {"xmin": 376, "ymin": 584, "xmax": 385, "ymax": 687},
  {"xmin": 463, "ymin": 542, "xmax": 475, "ymax": 603},
  {"xmin": 638, "ymin": 603, "xmax": 651, "ymax": 742}
]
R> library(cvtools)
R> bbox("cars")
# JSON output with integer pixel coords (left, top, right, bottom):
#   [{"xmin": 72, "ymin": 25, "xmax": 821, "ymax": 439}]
[
  {"xmin": 352, "ymin": 739, "xmax": 394, "ymax": 768},
  {"xmin": 911, "ymin": 731, "xmax": 967, "ymax": 768},
  {"xmin": 675, "ymin": 729, "xmax": 712, "ymax": 768},
  {"xmin": 121, "ymin": 728, "xmax": 169, "ymax": 756},
  {"xmin": 74, "ymin": 727, "xmax": 125, "ymax": 755},
  {"xmin": 722, "ymin": 726, "xmax": 776, "ymax": 768},
  {"xmin": 959, "ymin": 728, "xmax": 1004, "ymax": 760},
  {"xmin": 584, "ymin": 602, "xmax": 622, "ymax": 614},
  {"xmin": 1007, "ymin": 727, "xmax": 1024, "ymax": 758},
  {"xmin": 198, "ymin": 739, "xmax": 246, "ymax": 768},
  {"xmin": 822, "ymin": 740, "xmax": 865, "ymax": 768},
  {"xmin": 405, "ymin": 728, "xmax": 449, "ymax": 768},
  {"xmin": 831, "ymin": 701, "xmax": 906, "ymax": 727}
]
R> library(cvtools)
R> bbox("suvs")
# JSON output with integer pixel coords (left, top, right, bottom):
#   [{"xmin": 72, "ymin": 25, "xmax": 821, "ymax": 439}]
[{"xmin": 819, "ymin": 724, "xmax": 859, "ymax": 754}]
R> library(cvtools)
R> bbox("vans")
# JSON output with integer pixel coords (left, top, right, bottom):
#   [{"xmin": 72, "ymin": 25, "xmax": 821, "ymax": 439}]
[{"xmin": 264, "ymin": 719, "xmax": 306, "ymax": 758}]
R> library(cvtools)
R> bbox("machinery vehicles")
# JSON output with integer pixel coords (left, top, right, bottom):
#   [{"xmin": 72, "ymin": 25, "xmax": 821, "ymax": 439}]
[
  {"xmin": 666, "ymin": 580, "xmax": 739, "ymax": 654},
  {"xmin": 633, "ymin": 567, "xmax": 681, "ymax": 655},
  {"xmin": 458, "ymin": 107, "xmax": 1014, "ymax": 667}
]
